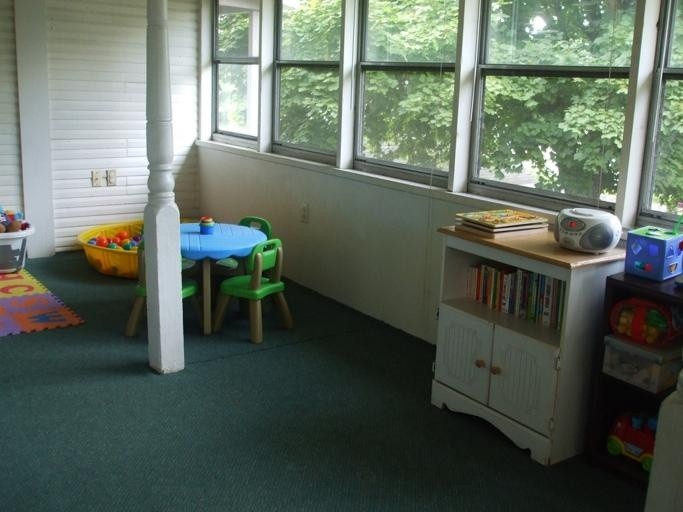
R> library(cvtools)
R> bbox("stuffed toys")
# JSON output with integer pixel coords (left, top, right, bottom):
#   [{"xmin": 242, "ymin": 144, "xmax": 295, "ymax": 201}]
[{"xmin": 0, "ymin": 207, "xmax": 30, "ymax": 233}]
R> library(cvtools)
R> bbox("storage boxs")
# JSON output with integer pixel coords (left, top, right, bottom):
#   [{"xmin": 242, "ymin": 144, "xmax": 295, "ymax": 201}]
[{"xmin": 596, "ymin": 223, "xmax": 683, "ymax": 482}]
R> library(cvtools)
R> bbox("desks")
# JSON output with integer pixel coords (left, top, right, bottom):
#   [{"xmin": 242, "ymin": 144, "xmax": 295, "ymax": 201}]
[{"xmin": 180, "ymin": 221, "xmax": 267, "ymax": 337}]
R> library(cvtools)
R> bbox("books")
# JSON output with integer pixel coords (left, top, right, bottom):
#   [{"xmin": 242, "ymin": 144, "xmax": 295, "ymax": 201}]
[{"xmin": 461, "ymin": 258, "xmax": 567, "ymax": 333}]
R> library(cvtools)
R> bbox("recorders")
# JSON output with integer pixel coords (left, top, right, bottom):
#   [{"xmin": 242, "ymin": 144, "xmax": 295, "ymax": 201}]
[{"xmin": 553, "ymin": 206, "xmax": 624, "ymax": 255}]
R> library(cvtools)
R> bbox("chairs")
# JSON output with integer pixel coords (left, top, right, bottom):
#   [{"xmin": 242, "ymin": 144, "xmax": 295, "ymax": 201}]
[
  {"xmin": 126, "ymin": 240, "xmax": 199, "ymax": 339},
  {"xmin": 141, "ymin": 226, "xmax": 196, "ymax": 275},
  {"xmin": 124, "ymin": 215, "xmax": 296, "ymax": 344},
  {"xmin": 212, "ymin": 238, "xmax": 294, "ymax": 344},
  {"xmin": 214, "ymin": 216, "xmax": 273, "ymax": 278}
]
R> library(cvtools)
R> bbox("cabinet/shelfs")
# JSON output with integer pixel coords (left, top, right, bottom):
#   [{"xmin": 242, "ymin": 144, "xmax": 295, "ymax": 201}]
[{"xmin": 428, "ymin": 224, "xmax": 627, "ymax": 465}]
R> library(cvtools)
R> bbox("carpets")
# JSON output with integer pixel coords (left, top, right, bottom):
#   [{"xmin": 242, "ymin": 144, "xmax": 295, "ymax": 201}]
[{"xmin": 0, "ymin": 266, "xmax": 86, "ymax": 337}]
